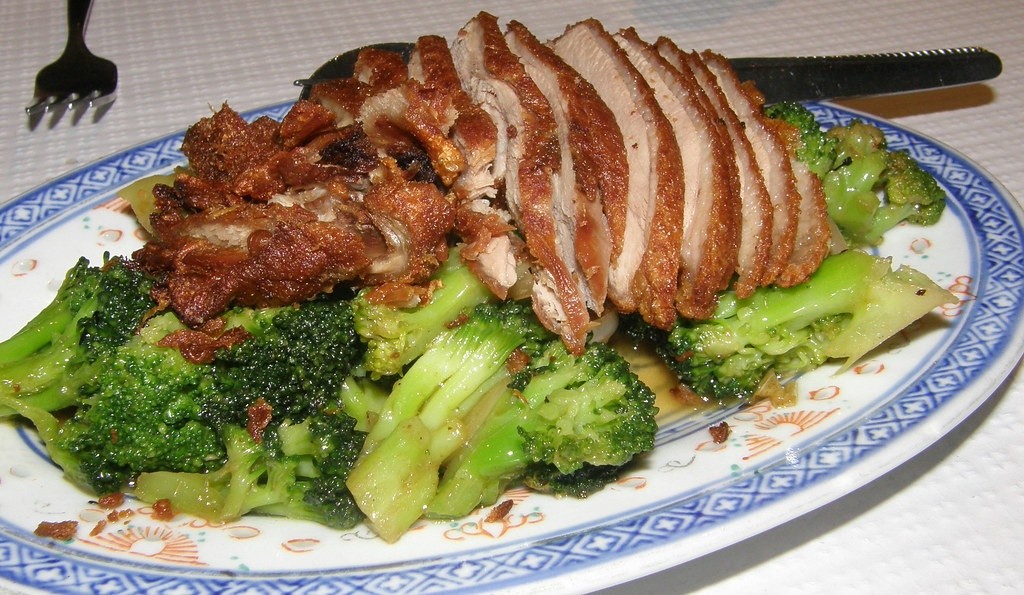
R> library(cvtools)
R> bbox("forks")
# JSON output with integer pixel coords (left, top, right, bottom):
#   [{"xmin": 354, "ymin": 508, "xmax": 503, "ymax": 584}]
[{"xmin": 24, "ymin": 0, "xmax": 118, "ymax": 114}]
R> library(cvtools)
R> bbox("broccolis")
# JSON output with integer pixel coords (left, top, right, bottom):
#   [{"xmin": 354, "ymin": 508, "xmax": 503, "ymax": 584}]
[{"xmin": 0, "ymin": 101, "xmax": 963, "ymax": 546}]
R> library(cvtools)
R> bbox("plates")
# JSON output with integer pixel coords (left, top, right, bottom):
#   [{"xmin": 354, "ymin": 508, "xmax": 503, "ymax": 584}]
[{"xmin": 0, "ymin": 99, "xmax": 1024, "ymax": 595}]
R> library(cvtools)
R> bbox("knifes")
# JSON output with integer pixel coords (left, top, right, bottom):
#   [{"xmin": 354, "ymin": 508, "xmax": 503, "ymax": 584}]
[{"xmin": 298, "ymin": 43, "xmax": 1004, "ymax": 103}]
